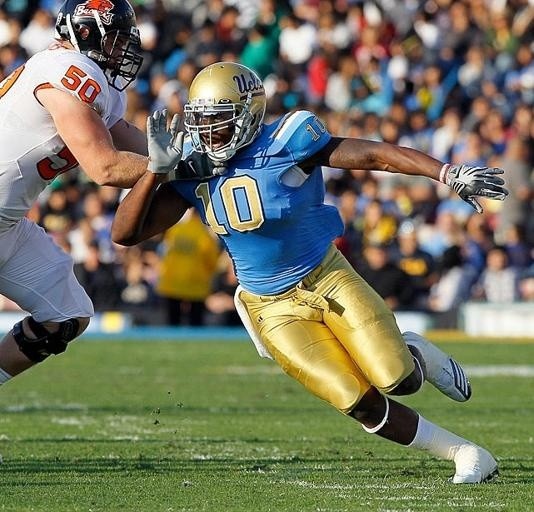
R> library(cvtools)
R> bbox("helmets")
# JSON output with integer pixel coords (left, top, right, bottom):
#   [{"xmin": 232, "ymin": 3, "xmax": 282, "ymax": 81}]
[
  {"xmin": 55, "ymin": 0, "xmax": 144, "ymax": 92},
  {"xmin": 182, "ymin": 61, "xmax": 268, "ymax": 163}
]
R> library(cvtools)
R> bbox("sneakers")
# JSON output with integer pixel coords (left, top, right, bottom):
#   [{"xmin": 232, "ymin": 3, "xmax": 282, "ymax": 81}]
[
  {"xmin": 404, "ymin": 330, "xmax": 473, "ymax": 403},
  {"xmin": 445, "ymin": 436, "xmax": 500, "ymax": 486}
]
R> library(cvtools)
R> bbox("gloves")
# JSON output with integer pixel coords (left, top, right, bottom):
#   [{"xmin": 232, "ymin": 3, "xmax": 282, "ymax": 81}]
[
  {"xmin": 439, "ymin": 163, "xmax": 509, "ymax": 214},
  {"xmin": 146, "ymin": 107, "xmax": 185, "ymax": 176}
]
